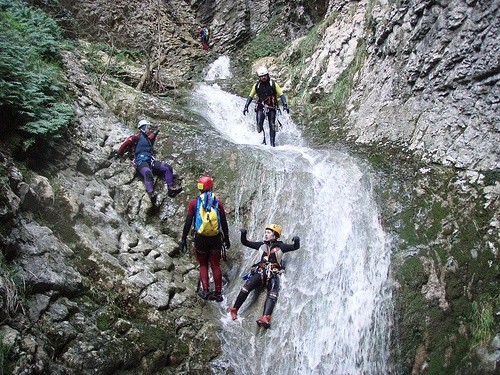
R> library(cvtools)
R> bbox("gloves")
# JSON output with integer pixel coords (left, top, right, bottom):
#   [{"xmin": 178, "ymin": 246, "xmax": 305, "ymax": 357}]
[
  {"xmin": 225, "ymin": 239, "xmax": 230, "ymax": 250},
  {"xmin": 292, "ymin": 236, "xmax": 301, "ymax": 244},
  {"xmin": 239, "ymin": 228, "xmax": 247, "ymax": 235},
  {"xmin": 242, "ymin": 104, "xmax": 248, "ymax": 116},
  {"xmin": 181, "ymin": 238, "xmax": 187, "ymax": 250},
  {"xmin": 283, "ymin": 103, "xmax": 289, "ymax": 114}
]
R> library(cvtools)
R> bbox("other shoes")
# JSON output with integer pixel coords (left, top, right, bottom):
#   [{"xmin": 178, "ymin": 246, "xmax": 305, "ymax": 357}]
[
  {"xmin": 169, "ymin": 185, "xmax": 182, "ymax": 198},
  {"xmin": 270, "ymin": 141, "xmax": 275, "ymax": 147},
  {"xmin": 151, "ymin": 192, "xmax": 157, "ymax": 203}
]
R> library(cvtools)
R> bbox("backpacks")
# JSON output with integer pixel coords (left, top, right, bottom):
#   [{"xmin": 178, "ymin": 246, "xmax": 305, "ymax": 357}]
[
  {"xmin": 194, "ymin": 191, "xmax": 225, "ymax": 239},
  {"xmin": 203, "ymin": 27, "xmax": 209, "ymax": 37}
]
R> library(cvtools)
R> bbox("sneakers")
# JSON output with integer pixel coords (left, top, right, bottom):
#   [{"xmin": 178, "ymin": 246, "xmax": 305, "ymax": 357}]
[
  {"xmin": 198, "ymin": 290, "xmax": 210, "ymax": 300},
  {"xmin": 210, "ymin": 291, "xmax": 223, "ymax": 302},
  {"xmin": 256, "ymin": 314, "xmax": 272, "ymax": 329},
  {"xmin": 231, "ymin": 308, "xmax": 238, "ymax": 320}
]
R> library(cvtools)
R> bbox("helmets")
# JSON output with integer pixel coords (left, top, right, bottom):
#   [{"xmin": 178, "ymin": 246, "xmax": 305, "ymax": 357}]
[
  {"xmin": 197, "ymin": 25, "xmax": 201, "ymax": 29},
  {"xmin": 137, "ymin": 120, "xmax": 152, "ymax": 129},
  {"xmin": 197, "ymin": 177, "xmax": 214, "ymax": 193},
  {"xmin": 265, "ymin": 223, "xmax": 282, "ymax": 238},
  {"xmin": 256, "ymin": 67, "xmax": 269, "ymax": 77}
]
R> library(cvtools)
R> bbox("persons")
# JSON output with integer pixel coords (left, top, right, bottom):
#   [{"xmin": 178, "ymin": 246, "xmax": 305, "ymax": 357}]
[
  {"xmin": 243, "ymin": 65, "xmax": 289, "ymax": 147},
  {"xmin": 230, "ymin": 224, "xmax": 301, "ymax": 328},
  {"xmin": 193, "ymin": 28, "xmax": 210, "ymax": 50},
  {"xmin": 175, "ymin": 176, "xmax": 231, "ymax": 302},
  {"xmin": 111, "ymin": 120, "xmax": 183, "ymax": 210}
]
